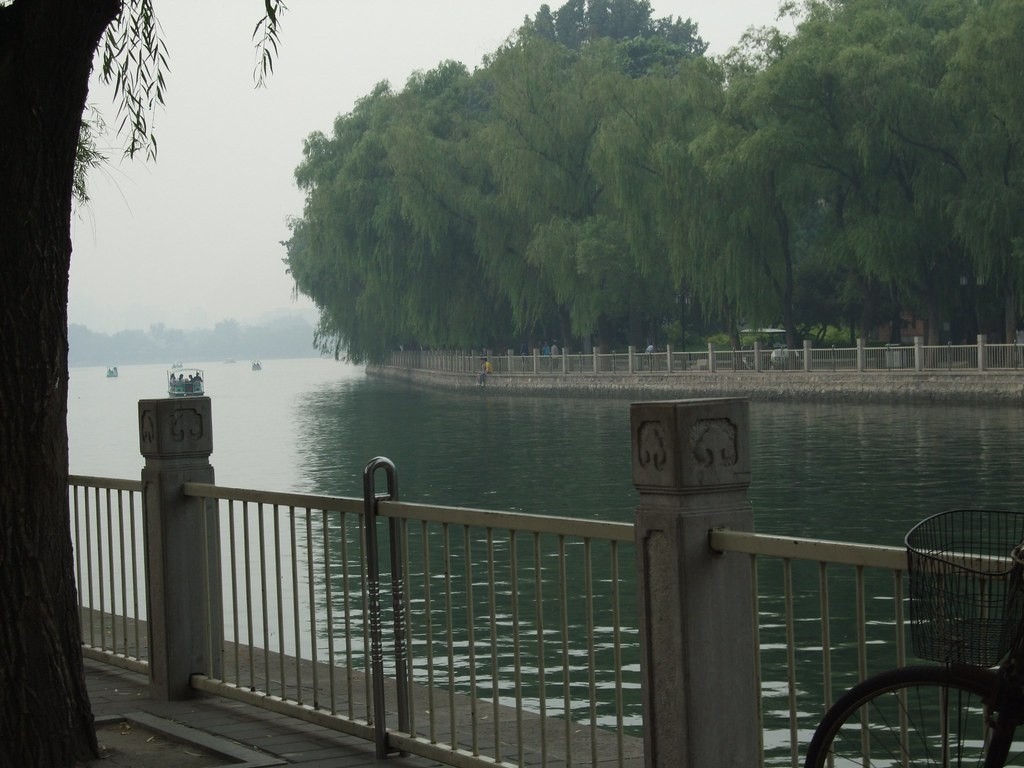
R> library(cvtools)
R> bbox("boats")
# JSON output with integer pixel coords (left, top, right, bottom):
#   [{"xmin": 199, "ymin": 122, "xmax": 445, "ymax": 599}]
[
  {"xmin": 251, "ymin": 361, "xmax": 261, "ymax": 371},
  {"xmin": 167, "ymin": 368, "xmax": 205, "ymax": 398},
  {"xmin": 106, "ymin": 366, "xmax": 118, "ymax": 378},
  {"xmin": 171, "ymin": 363, "xmax": 183, "ymax": 369}
]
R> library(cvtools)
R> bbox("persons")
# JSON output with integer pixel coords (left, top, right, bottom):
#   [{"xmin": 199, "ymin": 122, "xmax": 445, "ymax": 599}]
[
  {"xmin": 169, "ymin": 374, "xmax": 176, "ymax": 387},
  {"xmin": 188, "ymin": 375, "xmax": 194, "ymax": 383},
  {"xmin": 645, "ymin": 342, "xmax": 657, "ymax": 371},
  {"xmin": 477, "ymin": 357, "xmax": 493, "ymax": 387},
  {"xmin": 176, "ymin": 374, "xmax": 185, "ymax": 382},
  {"xmin": 520, "ymin": 340, "xmax": 562, "ymax": 373},
  {"xmin": 192, "ymin": 372, "xmax": 204, "ymax": 384}
]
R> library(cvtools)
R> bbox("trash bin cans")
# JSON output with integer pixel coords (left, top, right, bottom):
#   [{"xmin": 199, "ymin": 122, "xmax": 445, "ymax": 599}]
[{"xmin": 885, "ymin": 342, "xmax": 907, "ymax": 369}]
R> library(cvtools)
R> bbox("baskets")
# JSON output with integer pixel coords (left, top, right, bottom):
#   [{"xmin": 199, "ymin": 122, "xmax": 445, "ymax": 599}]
[{"xmin": 902, "ymin": 506, "xmax": 1024, "ymax": 668}]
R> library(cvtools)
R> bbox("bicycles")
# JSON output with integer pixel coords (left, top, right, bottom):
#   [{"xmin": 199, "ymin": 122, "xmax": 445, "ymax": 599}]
[{"xmin": 802, "ymin": 505, "xmax": 1023, "ymax": 768}]
